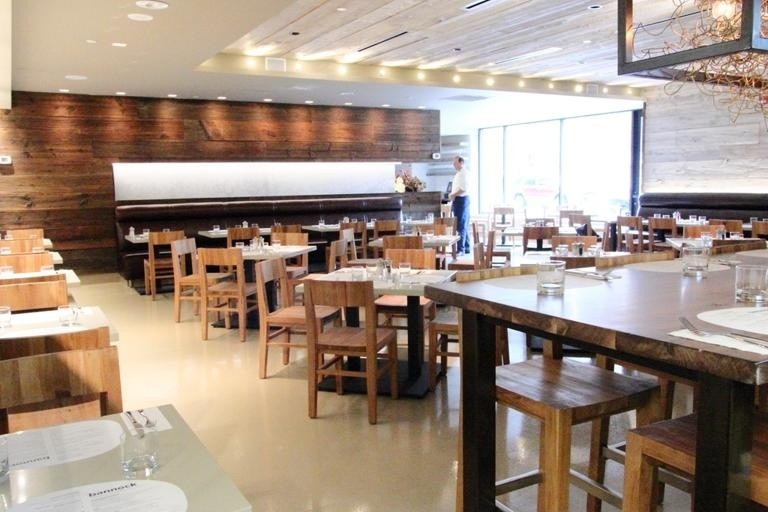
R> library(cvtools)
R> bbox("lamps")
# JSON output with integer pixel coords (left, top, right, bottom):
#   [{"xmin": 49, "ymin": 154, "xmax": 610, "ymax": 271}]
[{"xmin": 615, "ymin": 0, "xmax": 768, "ymax": 132}]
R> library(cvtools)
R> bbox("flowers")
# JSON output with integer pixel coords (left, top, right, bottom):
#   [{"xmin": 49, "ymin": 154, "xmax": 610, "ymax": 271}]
[{"xmin": 396, "ymin": 168, "xmax": 426, "ymax": 194}]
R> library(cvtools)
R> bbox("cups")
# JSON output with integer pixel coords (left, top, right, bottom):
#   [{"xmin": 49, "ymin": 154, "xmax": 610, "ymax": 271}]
[
  {"xmin": 350, "ymin": 265, "xmax": 363, "ymax": 281},
  {"xmin": 734, "ymin": 264, "xmax": 768, "ymax": 304},
  {"xmin": 426, "ymin": 229, "xmax": 434, "ymax": 240},
  {"xmin": 0, "ymin": 246, "xmax": 12, "ymax": 254},
  {"xmin": 428, "ymin": 212, "xmax": 433, "ymax": 222},
  {"xmin": 398, "ymin": 261, "xmax": 412, "ymax": 281},
  {"xmin": 699, "ymin": 232, "xmax": 712, "ymax": 247},
  {"xmin": 38, "ymin": 264, "xmax": 55, "ymax": 276},
  {"xmin": 142, "ymin": 227, "xmax": 150, "ymax": 237},
  {"xmin": 0, "ymin": 266, "xmax": 14, "ymax": 278},
  {"xmin": 536, "ymin": 260, "xmax": 566, "ymax": 298},
  {"xmin": 212, "ymin": 224, "xmax": 220, "ymax": 232},
  {"xmin": 698, "ymin": 215, "xmax": 707, "ymax": 226},
  {"xmin": 365, "ymin": 263, "xmax": 377, "ymax": 279},
  {"xmin": 559, "ymin": 244, "xmax": 568, "ymax": 257},
  {"xmin": 162, "ymin": 229, "xmax": 170, "ymax": 232},
  {"xmin": 271, "ymin": 239, "xmax": 281, "ymax": 253},
  {"xmin": 0, "ymin": 437, "xmax": 10, "ymax": 484},
  {"xmin": 587, "ymin": 247, "xmax": 597, "ymax": 256},
  {"xmin": 405, "ymin": 216, "xmax": 411, "ymax": 224},
  {"xmin": 31, "ymin": 245, "xmax": 44, "ymax": 254},
  {"xmin": 235, "ymin": 224, "xmax": 241, "ymax": 228},
  {"xmin": 680, "ymin": 224, "xmax": 714, "ymax": 276},
  {"xmin": 318, "ymin": 220, "xmax": 324, "ymax": 227},
  {"xmin": 729, "ymin": 231, "xmax": 739, "ymax": 241},
  {"xmin": 351, "ymin": 218, "xmax": 358, "ymax": 223},
  {"xmin": 4, "ymin": 234, "xmax": 14, "ymax": 240},
  {"xmin": 120, "ymin": 429, "xmax": 160, "ymax": 480},
  {"xmin": 58, "ymin": 304, "xmax": 79, "ymax": 327},
  {"xmin": 252, "ymin": 223, "xmax": 259, "ymax": 229},
  {"xmin": 0, "ymin": 306, "xmax": 13, "ymax": 328},
  {"xmin": 29, "ymin": 234, "xmax": 39, "ymax": 240},
  {"xmin": 750, "ymin": 216, "xmax": 758, "ymax": 225},
  {"xmin": 235, "ymin": 242, "xmax": 244, "ymax": 249},
  {"xmin": 371, "ymin": 218, "xmax": 377, "ymax": 227},
  {"xmin": 689, "ymin": 214, "xmax": 697, "ymax": 224}
]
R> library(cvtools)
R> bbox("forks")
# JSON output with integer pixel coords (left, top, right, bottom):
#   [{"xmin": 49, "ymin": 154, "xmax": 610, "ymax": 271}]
[
  {"xmin": 567, "ymin": 267, "xmax": 614, "ymax": 277},
  {"xmin": 677, "ymin": 315, "xmax": 767, "ymax": 350}
]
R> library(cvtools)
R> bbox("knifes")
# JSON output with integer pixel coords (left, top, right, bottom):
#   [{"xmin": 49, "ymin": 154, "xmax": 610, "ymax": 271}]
[{"xmin": 685, "ymin": 314, "xmax": 768, "ymax": 343}]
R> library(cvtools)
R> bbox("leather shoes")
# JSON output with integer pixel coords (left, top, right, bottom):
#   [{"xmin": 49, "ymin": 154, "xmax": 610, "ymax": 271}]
[
  {"xmin": 456, "ymin": 252, "xmax": 465, "ymax": 256},
  {"xmin": 465, "ymin": 251, "xmax": 470, "ymax": 254}
]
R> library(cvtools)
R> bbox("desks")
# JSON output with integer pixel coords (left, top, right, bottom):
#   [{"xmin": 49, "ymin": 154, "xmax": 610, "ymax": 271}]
[
  {"xmin": 44, "ymin": 237, "xmax": 53, "ymax": 250},
  {"xmin": 226, "ymin": 244, "xmax": 317, "ymax": 263},
  {"xmin": 549, "ymin": 249, "xmax": 674, "ymax": 271},
  {"xmin": 0, "ymin": 267, "xmax": 81, "ymax": 284},
  {"xmin": 324, "ymin": 265, "xmax": 461, "ymax": 384},
  {"xmin": 48, "ymin": 250, "xmax": 64, "ymax": 266},
  {"xmin": 642, "ymin": 217, "xmax": 709, "ymax": 229},
  {"xmin": 742, "ymin": 221, "xmax": 768, "ymax": 232},
  {"xmin": 422, "ymin": 253, "xmax": 768, "ymax": 512},
  {"xmin": 0, "ymin": 402, "xmax": 255, "ymax": 512},
  {"xmin": 662, "ymin": 236, "xmax": 766, "ymax": 258},
  {"xmin": 522, "ymin": 226, "xmax": 560, "ymax": 255},
  {"xmin": 0, "ymin": 305, "xmax": 112, "ymax": 358},
  {"xmin": 301, "ymin": 222, "xmax": 340, "ymax": 234},
  {"xmin": 367, "ymin": 233, "xmax": 462, "ymax": 249},
  {"xmin": 194, "ymin": 226, "xmax": 274, "ymax": 240},
  {"xmin": 123, "ymin": 231, "xmax": 153, "ymax": 247}
]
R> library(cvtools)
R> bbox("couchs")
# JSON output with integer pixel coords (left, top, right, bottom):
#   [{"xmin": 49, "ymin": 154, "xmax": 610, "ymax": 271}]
[
  {"xmin": 637, "ymin": 192, "xmax": 768, "ymax": 223},
  {"xmin": 112, "ymin": 192, "xmax": 404, "ymax": 281}
]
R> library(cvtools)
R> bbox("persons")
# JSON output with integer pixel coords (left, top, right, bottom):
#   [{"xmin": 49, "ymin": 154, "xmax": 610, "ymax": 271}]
[{"xmin": 449, "ymin": 157, "xmax": 473, "ymax": 256}]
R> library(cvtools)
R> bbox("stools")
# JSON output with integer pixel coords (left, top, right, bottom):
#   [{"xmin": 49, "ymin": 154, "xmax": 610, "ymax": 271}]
[
  {"xmin": 454, "ymin": 355, "xmax": 662, "ymax": 512},
  {"xmin": 587, "ymin": 352, "xmax": 703, "ymax": 512},
  {"xmin": 621, "ymin": 403, "xmax": 767, "ymax": 512}
]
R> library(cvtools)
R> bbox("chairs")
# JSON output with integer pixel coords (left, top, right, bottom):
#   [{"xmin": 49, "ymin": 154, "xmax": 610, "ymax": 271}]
[
  {"xmin": 340, "ymin": 228, "xmax": 357, "ymax": 261},
  {"xmin": 569, "ymin": 214, "xmax": 592, "ymax": 237},
  {"xmin": 143, "ymin": 229, "xmax": 186, "ymax": 301},
  {"xmin": 751, "ymin": 220, "xmax": 768, "ymax": 238},
  {"xmin": 195, "ymin": 247, "xmax": 259, "ymax": 343},
  {"xmin": 373, "ymin": 219, "xmax": 400, "ymax": 239},
  {"xmin": 492, "ymin": 205, "xmax": 516, "ymax": 247},
  {"xmin": 708, "ymin": 218, "xmax": 744, "ymax": 237},
  {"xmin": 5, "ymin": 228, "xmax": 44, "ymax": 240},
  {"xmin": 601, "ymin": 220, "xmax": 610, "ymax": 251},
  {"xmin": 0, "ymin": 345, "xmax": 124, "ymax": 439},
  {"xmin": 682, "ymin": 224, "xmax": 726, "ymax": 239},
  {"xmin": 339, "ymin": 221, "xmax": 368, "ymax": 259},
  {"xmin": 551, "ymin": 235, "xmax": 599, "ymax": 253},
  {"xmin": 375, "ymin": 247, "xmax": 438, "ymax": 359},
  {"xmin": 648, "ymin": 216, "xmax": 677, "ymax": 254},
  {"xmin": 0, "ymin": 279, "xmax": 69, "ymax": 317},
  {"xmin": 1, "ymin": 235, "xmax": 45, "ymax": 254},
  {"xmin": 473, "ymin": 222, "xmax": 512, "ymax": 265},
  {"xmin": 254, "ymin": 256, "xmax": 349, "ymax": 381},
  {"xmin": 512, "ymin": 208, "xmax": 528, "ymax": 246},
  {"xmin": 447, "ymin": 230, "xmax": 496, "ymax": 270},
  {"xmin": 302, "ymin": 277, "xmax": 402, "ymax": 425},
  {"xmin": 220, "ymin": 226, "xmax": 261, "ymax": 281},
  {"xmin": 524, "ymin": 217, "xmax": 555, "ymax": 227},
  {"xmin": 270, "ymin": 231, "xmax": 310, "ymax": 308},
  {"xmin": 0, "ymin": 252, "xmax": 56, "ymax": 275},
  {"xmin": 617, "ymin": 215, "xmax": 643, "ymax": 254},
  {"xmin": 559, "ymin": 209, "xmax": 584, "ymax": 230},
  {"xmin": 381, "ymin": 234, "xmax": 423, "ymax": 252},
  {"xmin": 269, "ymin": 223, "xmax": 304, "ymax": 236},
  {"xmin": 434, "ymin": 216, "xmax": 458, "ymax": 261},
  {"xmin": 473, "ymin": 241, "xmax": 485, "ymax": 269},
  {"xmin": 328, "ymin": 239, "xmax": 347, "ymax": 274},
  {"xmin": 169, "ymin": 238, "xmax": 234, "ymax": 324}
]
